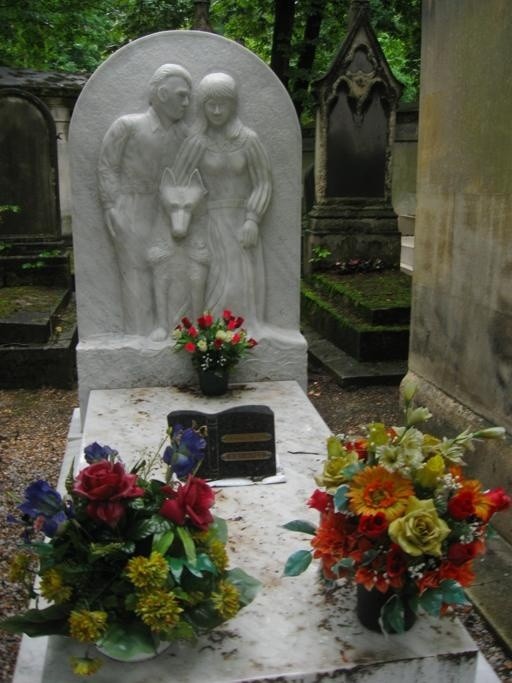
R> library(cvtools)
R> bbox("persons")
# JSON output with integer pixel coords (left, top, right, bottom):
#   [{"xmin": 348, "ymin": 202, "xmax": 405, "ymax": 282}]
[
  {"xmin": 96, "ymin": 61, "xmax": 195, "ymax": 350},
  {"xmin": 165, "ymin": 69, "xmax": 280, "ymax": 323}
]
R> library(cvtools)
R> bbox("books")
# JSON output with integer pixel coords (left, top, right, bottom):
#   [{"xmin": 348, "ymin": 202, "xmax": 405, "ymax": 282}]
[{"xmin": 164, "ymin": 403, "xmax": 278, "ymax": 483}]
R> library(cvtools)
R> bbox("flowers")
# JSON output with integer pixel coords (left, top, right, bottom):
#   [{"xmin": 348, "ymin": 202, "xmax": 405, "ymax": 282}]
[
  {"xmin": 278, "ymin": 370, "xmax": 512, "ymax": 641},
  {"xmin": 171, "ymin": 309, "xmax": 257, "ymax": 379},
  {"xmin": 0, "ymin": 422, "xmax": 264, "ymax": 677}
]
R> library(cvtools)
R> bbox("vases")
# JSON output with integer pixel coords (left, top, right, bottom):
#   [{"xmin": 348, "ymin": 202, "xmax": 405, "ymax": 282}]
[
  {"xmin": 195, "ymin": 367, "xmax": 230, "ymax": 396},
  {"xmin": 94, "ymin": 619, "xmax": 172, "ymax": 662},
  {"xmin": 356, "ymin": 583, "xmax": 416, "ymax": 634}
]
